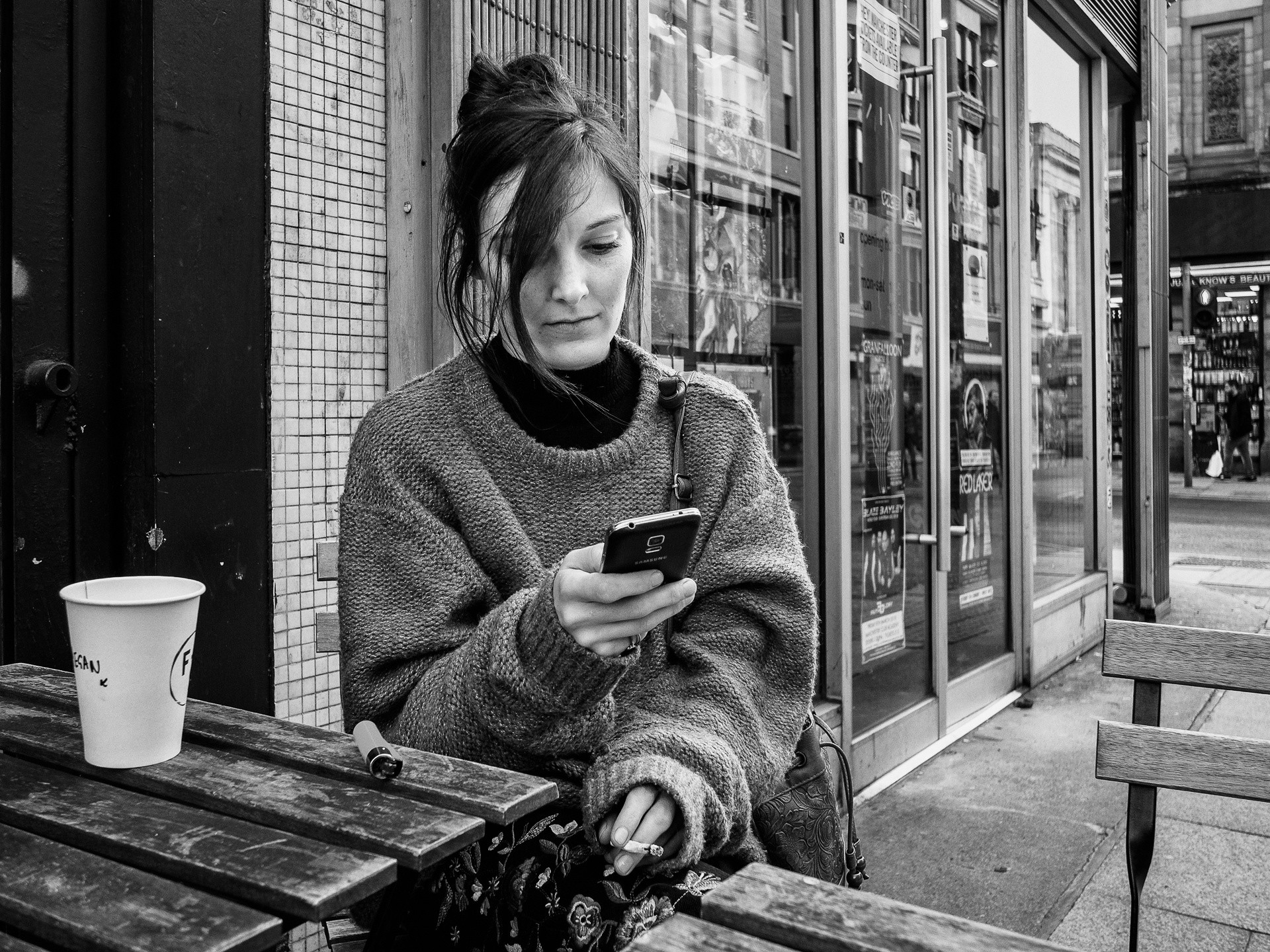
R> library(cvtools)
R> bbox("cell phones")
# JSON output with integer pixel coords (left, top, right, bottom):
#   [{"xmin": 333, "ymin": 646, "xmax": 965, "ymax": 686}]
[{"xmin": 600, "ymin": 506, "xmax": 702, "ymax": 586}]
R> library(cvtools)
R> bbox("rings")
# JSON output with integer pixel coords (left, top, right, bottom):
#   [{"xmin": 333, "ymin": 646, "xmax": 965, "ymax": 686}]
[{"xmin": 627, "ymin": 634, "xmax": 641, "ymax": 651}]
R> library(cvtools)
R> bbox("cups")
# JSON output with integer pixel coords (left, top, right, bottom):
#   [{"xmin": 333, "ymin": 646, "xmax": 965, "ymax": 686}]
[{"xmin": 59, "ymin": 576, "xmax": 206, "ymax": 769}]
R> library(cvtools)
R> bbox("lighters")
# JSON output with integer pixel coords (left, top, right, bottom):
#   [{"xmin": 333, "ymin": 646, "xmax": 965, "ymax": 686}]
[{"xmin": 353, "ymin": 720, "xmax": 403, "ymax": 779}]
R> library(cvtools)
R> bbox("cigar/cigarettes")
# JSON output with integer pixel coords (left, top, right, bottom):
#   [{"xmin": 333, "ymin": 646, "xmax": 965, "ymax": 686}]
[{"xmin": 610, "ymin": 840, "xmax": 664, "ymax": 857}]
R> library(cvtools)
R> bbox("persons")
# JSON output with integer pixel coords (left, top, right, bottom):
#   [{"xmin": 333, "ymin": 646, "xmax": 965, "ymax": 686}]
[
  {"xmin": 1216, "ymin": 379, "xmax": 1257, "ymax": 482},
  {"xmin": 339, "ymin": 51, "xmax": 820, "ymax": 879}
]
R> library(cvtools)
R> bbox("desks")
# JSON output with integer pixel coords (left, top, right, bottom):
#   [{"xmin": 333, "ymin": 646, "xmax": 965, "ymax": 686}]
[
  {"xmin": 605, "ymin": 864, "xmax": 1082, "ymax": 952},
  {"xmin": 0, "ymin": 661, "xmax": 562, "ymax": 952}
]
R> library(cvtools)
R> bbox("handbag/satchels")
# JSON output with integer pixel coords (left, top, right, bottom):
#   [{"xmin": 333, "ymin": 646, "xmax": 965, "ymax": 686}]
[{"xmin": 669, "ymin": 370, "xmax": 846, "ymax": 889}]
